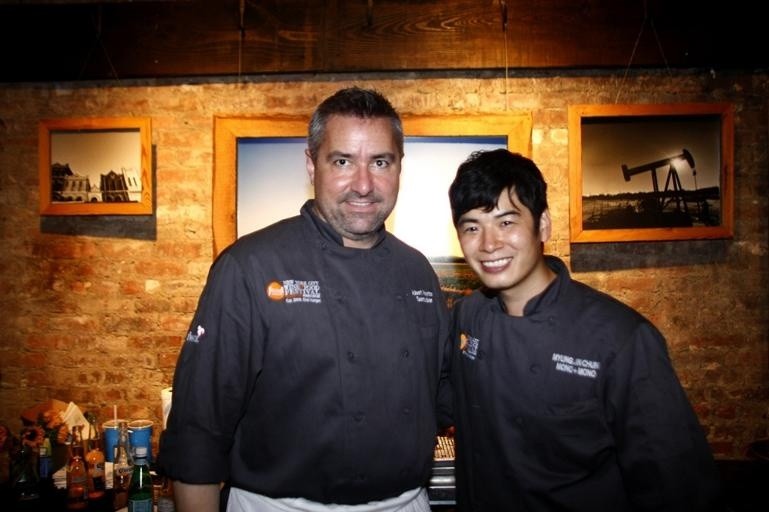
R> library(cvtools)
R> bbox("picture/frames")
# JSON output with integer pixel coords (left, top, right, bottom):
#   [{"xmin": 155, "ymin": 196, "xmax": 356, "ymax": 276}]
[
  {"xmin": 37, "ymin": 117, "xmax": 153, "ymax": 216},
  {"xmin": 567, "ymin": 102, "xmax": 735, "ymax": 245},
  {"xmin": 213, "ymin": 114, "xmax": 532, "ymax": 310}
]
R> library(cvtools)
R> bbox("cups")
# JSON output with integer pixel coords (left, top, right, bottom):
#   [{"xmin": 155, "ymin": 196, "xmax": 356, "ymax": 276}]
[
  {"xmin": 101, "ymin": 418, "xmax": 153, "ymax": 462},
  {"xmin": 38, "ymin": 453, "xmax": 53, "ymax": 478}
]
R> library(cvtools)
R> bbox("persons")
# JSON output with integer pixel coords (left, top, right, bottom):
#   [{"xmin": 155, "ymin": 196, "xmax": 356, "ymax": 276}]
[
  {"xmin": 149, "ymin": 87, "xmax": 453, "ymax": 511},
  {"xmin": 439, "ymin": 148, "xmax": 720, "ymax": 511}
]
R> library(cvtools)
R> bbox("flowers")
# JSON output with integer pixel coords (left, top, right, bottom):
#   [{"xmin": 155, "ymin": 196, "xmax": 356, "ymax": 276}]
[{"xmin": 0, "ymin": 406, "xmax": 68, "ymax": 448}]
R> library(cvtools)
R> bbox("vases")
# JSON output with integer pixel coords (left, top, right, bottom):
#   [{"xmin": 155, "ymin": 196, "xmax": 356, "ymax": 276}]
[{"xmin": 9, "ymin": 451, "xmax": 41, "ymax": 500}]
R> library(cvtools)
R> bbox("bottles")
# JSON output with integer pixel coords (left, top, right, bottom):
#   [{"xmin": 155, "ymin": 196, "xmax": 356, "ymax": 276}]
[
  {"xmin": 128, "ymin": 446, "xmax": 154, "ymax": 512},
  {"xmin": 110, "ymin": 421, "xmax": 129, "ymax": 493},
  {"xmin": 68, "ymin": 414, "xmax": 105, "ymax": 498}
]
